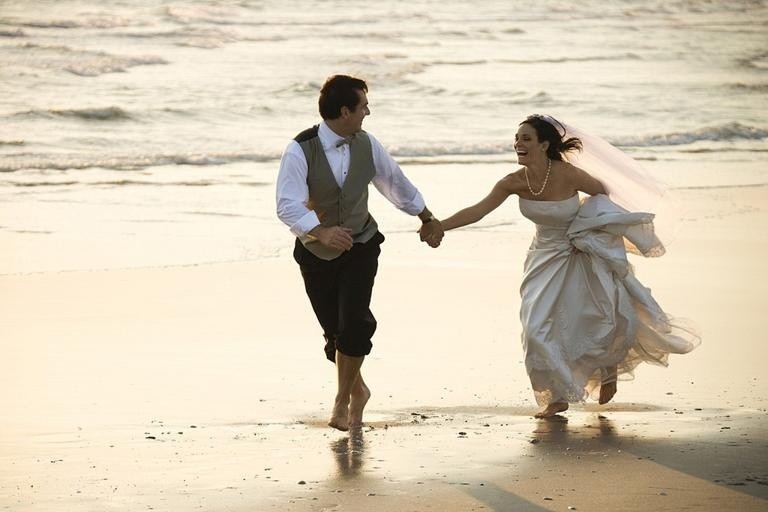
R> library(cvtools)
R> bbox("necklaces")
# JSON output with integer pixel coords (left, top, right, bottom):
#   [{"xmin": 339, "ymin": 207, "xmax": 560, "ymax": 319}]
[{"xmin": 524, "ymin": 158, "xmax": 552, "ymax": 197}]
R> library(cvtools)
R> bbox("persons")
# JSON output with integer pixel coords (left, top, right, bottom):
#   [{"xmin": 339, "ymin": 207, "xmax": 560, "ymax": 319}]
[
  {"xmin": 416, "ymin": 113, "xmax": 709, "ymax": 418},
  {"xmin": 276, "ymin": 76, "xmax": 444, "ymax": 431}
]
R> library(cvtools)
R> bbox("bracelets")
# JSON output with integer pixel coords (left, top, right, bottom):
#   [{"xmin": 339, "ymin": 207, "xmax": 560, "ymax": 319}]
[{"xmin": 423, "ymin": 215, "xmax": 435, "ymax": 222}]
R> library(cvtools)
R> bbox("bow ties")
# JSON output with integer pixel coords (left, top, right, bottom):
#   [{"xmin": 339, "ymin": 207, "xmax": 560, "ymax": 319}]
[{"xmin": 336, "ymin": 135, "xmax": 352, "ymax": 148}]
